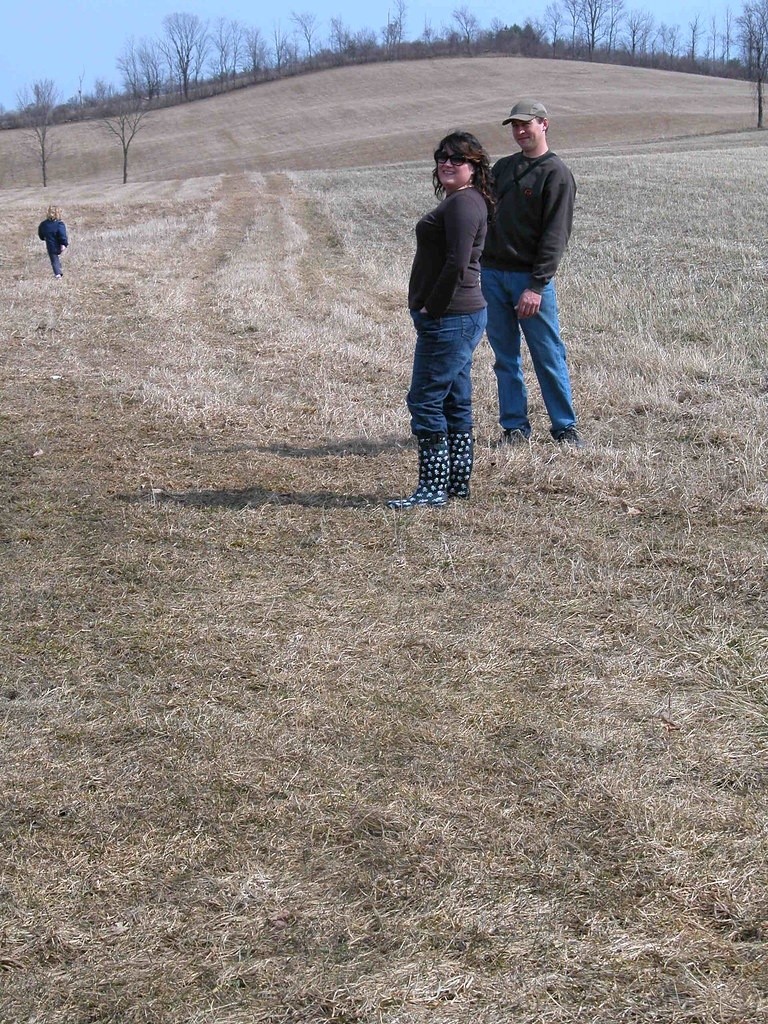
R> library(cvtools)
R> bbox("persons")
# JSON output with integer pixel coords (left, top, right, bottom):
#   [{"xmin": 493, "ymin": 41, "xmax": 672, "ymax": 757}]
[
  {"xmin": 38, "ymin": 204, "xmax": 69, "ymax": 279},
  {"xmin": 476, "ymin": 97, "xmax": 579, "ymax": 444},
  {"xmin": 386, "ymin": 132, "xmax": 497, "ymax": 510}
]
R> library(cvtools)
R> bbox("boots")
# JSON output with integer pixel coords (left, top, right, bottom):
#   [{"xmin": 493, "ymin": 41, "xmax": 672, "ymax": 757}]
[
  {"xmin": 385, "ymin": 434, "xmax": 449, "ymax": 511},
  {"xmin": 444, "ymin": 429, "xmax": 474, "ymax": 499}
]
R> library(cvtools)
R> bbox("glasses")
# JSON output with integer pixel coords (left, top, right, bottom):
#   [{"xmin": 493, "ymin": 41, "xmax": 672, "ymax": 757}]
[{"xmin": 434, "ymin": 149, "xmax": 473, "ymax": 166}]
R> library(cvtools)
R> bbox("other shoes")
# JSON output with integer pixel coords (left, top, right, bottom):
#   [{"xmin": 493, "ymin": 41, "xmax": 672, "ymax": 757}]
[{"xmin": 54, "ymin": 274, "xmax": 62, "ymax": 279}]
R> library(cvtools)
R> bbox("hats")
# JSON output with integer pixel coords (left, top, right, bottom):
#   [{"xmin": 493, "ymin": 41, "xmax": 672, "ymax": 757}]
[{"xmin": 501, "ymin": 100, "xmax": 547, "ymax": 126}]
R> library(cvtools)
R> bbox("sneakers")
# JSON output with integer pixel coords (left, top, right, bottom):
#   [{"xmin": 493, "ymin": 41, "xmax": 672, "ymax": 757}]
[
  {"xmin": 498, "ymin": 428, "xmax": 527, "ymax": 443},
  {"xmin": 559, "ymin": 421, "xmax": 579, "ymax": 448}
]
